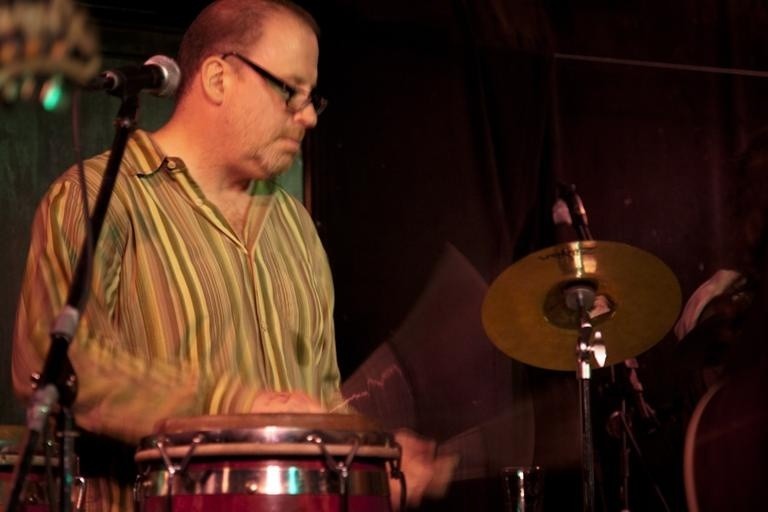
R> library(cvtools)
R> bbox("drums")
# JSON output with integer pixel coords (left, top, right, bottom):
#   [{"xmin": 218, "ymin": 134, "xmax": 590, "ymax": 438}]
[
  {"xmin": 131, "ymin": 427, "xmax": 406, "ymax": 510},
  {"xmin": 0, "ymin": 443, "xmax": 86, "ymax": 511}
]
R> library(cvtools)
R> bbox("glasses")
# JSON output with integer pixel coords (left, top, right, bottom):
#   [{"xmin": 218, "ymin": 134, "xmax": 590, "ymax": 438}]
[{"xmin": 222, "ymin": 51, "xmax": 328, "ymax": 116}]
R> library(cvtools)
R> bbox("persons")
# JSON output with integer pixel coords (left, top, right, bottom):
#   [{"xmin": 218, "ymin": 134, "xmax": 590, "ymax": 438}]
[{"xmin": 11, "ymin": 0, "xmax": 453, "ymax": 512}]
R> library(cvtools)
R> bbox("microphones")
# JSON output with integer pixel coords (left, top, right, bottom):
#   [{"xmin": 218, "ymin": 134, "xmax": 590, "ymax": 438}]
[{"xmin": 83, "ymin": 54, "xmax": 180, "ymax": 97}]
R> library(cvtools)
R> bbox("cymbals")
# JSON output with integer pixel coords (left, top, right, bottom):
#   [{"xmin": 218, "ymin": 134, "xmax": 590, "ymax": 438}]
[{"xmin": 482, "ymin": 239, "xmax": 682, "ymax": 372}]
